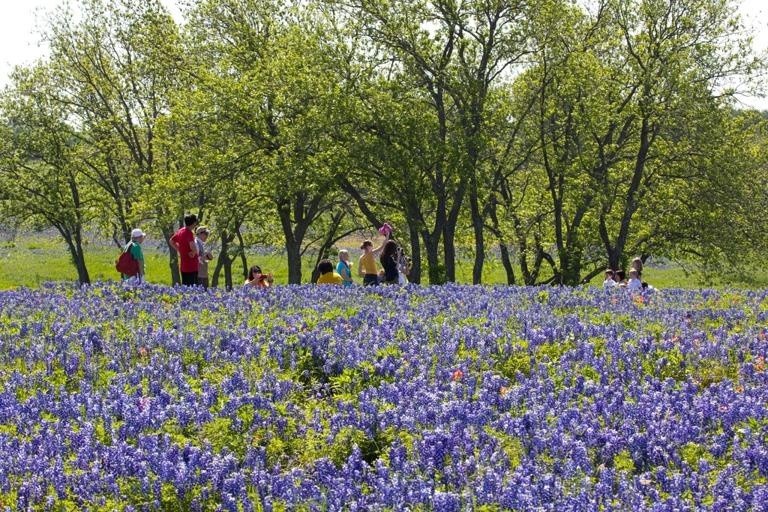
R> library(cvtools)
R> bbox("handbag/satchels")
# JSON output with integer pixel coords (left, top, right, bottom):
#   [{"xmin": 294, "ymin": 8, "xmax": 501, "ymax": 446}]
[{"xmin": 116, "ymin": 252, "xmax": 139, "ymax": 275}]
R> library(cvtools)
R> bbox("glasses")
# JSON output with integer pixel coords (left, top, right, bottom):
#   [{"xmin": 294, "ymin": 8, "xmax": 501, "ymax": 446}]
[{"xmin": 253, "ymin": 270, "xmax": 261, "ymax": 273}]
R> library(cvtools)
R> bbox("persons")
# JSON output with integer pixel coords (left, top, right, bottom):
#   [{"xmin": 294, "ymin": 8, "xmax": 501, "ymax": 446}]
[
  {"xmin": 169, "ymin": 214, "xmax": 199, "ymax": 286},
  {"xmin": 358, "ymin": 230, "xmax": 390, "ymax": 286},
  {"xmin": 194, "ymin": 226, "xmax": 213, "ymax": 291},
  {"xmin": 336, "ymin": 249, "xmax": 354, "ymax": 284},
  {"xmin": 243, "ymin": 265, "xmax": 273, "ymax": 287},
  {"xmin": 116, "ymin": 228, "xmax": 146, "ymax": 283},
  {"xmin": 378, "ymin": 240, "xmax": 411, "ymax": 283},
  {"xmin": 316, "ymin": 258, "xmax": 343, "ymax": 284},
  {"xmin": 603, "ymin": 257, "xmax": 652, "ymax": 290}
]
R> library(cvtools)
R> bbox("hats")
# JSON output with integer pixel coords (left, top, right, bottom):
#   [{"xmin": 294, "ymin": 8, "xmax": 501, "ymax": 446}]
[
  {"xmin": 131, "ymin": 229, "xmax": 146, "ymax": 237},
  {"xmin": 379, "ymin": 224, "xmax": 391, "ymax": 233},
  {"xmin": 185, "ymin": 214, "xmax": 198, "ymax": 225},
  {"xmin": 361, "ymin": 241, "xmax": 373, "ymax": 249},
  {"xmin": 196, "ymin": 226, "xmax": 210, "ymax": 233}
]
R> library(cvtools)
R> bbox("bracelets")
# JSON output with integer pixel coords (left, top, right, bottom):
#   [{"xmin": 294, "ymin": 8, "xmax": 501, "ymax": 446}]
[{"xmin": 268, "ymin": 279, "xmax": 273, "ymax": 282}]
